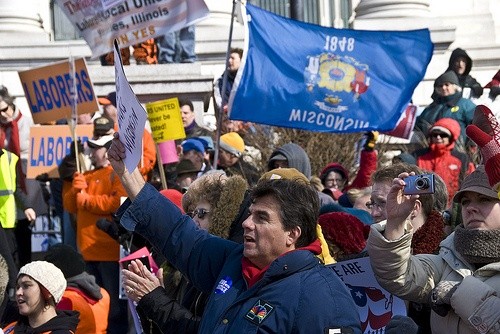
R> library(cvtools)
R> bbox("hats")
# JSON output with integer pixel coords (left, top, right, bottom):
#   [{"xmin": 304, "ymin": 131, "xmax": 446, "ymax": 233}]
[
  {"xmin": 435, "ymin": 71, "xmax": 458, "ymax": 86},
  {"xmin": 257, "ymin": 168, "xmax": 309, "ymax": 187},
  {"xmin": 18, "ymin": 260, "xmax": 68, "ymax": 304},
  {"xmin": 219, "ymin": 131, "xmax": 244, "ymax": 158},
  {"xmin": 270, "ymin": 153, "xmax": 287, "ymax": 162},
  {"xmin": 87, "ymin": 135, "xmax": 114, "ymax": 149},
  {"xmin": 93, "ymin": 116, "xmax": 114, "ymax": 131},
  {"xmin": 182, "ymin": 170, "xmax": 248, "ymax": 239},
  {"xmin": 453, "ymin": 164, "xmax": 500, "ymax": 203},
  {"xmin": 43, "ymin": 244, "xmax": 86, "ymax": 278},
  {"xmin": 181, "ymin": 139, "xmax": 205, "ymax": 154},
  {"xmin": 172, "ymin": 159, "xmax": 201, "ymax": 175},
  {"xmin": 192, "ymin": 137, "xmax": 212, "ymax": 149},
  {"xmin": 318, "ymin": 211, "xmax": 371, "ymax": 256}
]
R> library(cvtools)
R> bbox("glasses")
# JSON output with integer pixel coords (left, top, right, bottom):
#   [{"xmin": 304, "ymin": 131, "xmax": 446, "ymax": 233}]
[
  {"xmin": 430, "ymin": 132, "xmax": 449, "ymax": 139},
  {"xmin": 365, "ymin": 199, "xmax": 386, "ymax": 211},
  {"xmin": 185, "ymin": 208, "xmax": 210, "ymax": 218},
  {"xmin": 0, "ymin": 105, "xmax": 9, "ymax": 115}
]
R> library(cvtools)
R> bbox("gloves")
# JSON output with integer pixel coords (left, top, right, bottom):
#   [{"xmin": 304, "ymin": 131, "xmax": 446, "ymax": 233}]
[
  {"xmin": 76, "ymin": 192, "xmax": 88, "ymax": 210},
  {"xmin": 471, "ymin": 82, "xmax": 483, "ymax": 97},
  {"xmin": 490, "ymin": 86, "xmax": 500, "ymax": 97},
  {"xmin": 466, "ymin": 105, "xmax": 500, "ymax": 190},
  {"xmin": 72, "ymin": 171, "xmax": 88, "ymax": 190},
  {"xmin": 363, "ymin": 130, "xmax": 379, "ymax": 150},
  {"xmin": 385, "ymin": 315, "xmax": 418, "ymax": 334},
  {"xmin": 429, "ymin": 280, "xmax": 460, "ymax": 317}
]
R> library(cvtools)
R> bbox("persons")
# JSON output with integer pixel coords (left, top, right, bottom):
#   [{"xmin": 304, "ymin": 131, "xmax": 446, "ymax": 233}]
[
  {"xmin": 175, "ymin": 98, "xmax": 216, "ymax": 149},
  {"xmin": 0, "ymin": 261, "xmax": 81, "ymax": 334},
  {"xmin": 154, "ymin": 26, "xmax": 197, "ymax": 64},
  {"xmin": 107, "ymin": 131, "xmax": 362, "ymax": 334},
  {"xmin": 212, "ymin": 48, "xmax": 243, "ymax": 116},
  {"xmin": 420, "ymin": 71, "xmax": 477, "ymax": 158},
  {"xmin": 37, "ymin": 91, "xmax": 156, "ymax": 334},
  {"xmin": 0, "ymin": 147, "xmax": 36, "ymax": 306},
  {"xmin": 101, "ymin": 46, "xmax": 130, "ymax": 66},
  {"xmin": 0, "ymin": 84, "xmax": 22, "ymax": 156},
  {"xmin": 431, "ymin": 47, "xmax": 483, "ymax": 101},
  {"xmin": 125, "ymin": 130, "xmax": 380, "ymax": 334},
  {"xmin": 320, "ymin": 105, "xmax": 500, "ymax": 334},
  {"xmin": 133, "ymin": 38, "xmax": 158, "ymax": 64}
]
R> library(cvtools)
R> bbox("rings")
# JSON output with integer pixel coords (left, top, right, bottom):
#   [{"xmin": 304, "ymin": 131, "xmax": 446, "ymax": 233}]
[{"xmin": 126, "ymin": 288, "xmax": 132, "ymax": 293}]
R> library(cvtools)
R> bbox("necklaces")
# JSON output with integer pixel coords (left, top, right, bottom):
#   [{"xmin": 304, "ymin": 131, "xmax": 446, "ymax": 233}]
[{"xmin": 91, "ymin": 170, "xmax": 100, "ymax": 183}]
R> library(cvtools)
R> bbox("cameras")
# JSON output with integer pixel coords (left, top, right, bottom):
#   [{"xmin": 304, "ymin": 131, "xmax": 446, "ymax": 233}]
[{"xmin": 403, "ymin": 173, "xmax": 436, "ymax": 195}]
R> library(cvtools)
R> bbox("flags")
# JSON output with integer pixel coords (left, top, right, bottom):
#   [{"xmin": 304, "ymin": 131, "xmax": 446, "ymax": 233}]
[{"xmin": 227, "ymin": 3, "xmax": 434, "ymax": 133}]
